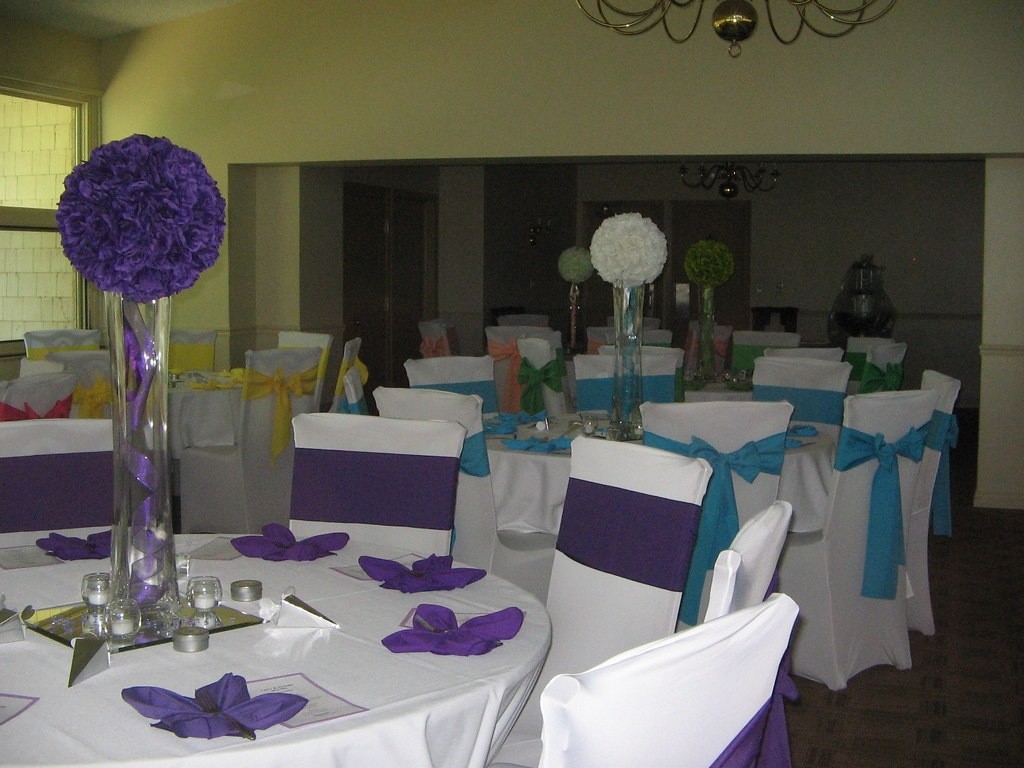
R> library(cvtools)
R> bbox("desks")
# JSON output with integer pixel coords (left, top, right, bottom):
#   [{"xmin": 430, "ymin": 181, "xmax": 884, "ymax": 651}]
[
  {"xmin": 471, "ymin": 409, "xmax": 841, "ymax": 535},
  {"xmin": 0, "ymin": 518, "xmax": 551, "ymax": 767}
]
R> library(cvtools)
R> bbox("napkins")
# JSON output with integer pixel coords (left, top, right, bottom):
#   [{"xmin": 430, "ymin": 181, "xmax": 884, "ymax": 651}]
[
  {"xmin": 121, "ymin": 673, "xmax": 308, "ymax": 742},
  {"xmin": 501, "ymin": 437, "xmax": 574, "ymax": 452},
  {"xmin": 36, "ymin": 526, "xmax": 114, "ymax": 561},
  {"xmin": 380, "ymin": 602, "xmax": 524, "ymax": 657},
  {"xmin": 230, "ymin": 522, "xmax": 350, "ymax": 564},
  {"xmin": 493, "ymin": 410, "xmax": 552, "ymax": 421},
  {"xmin": 482, "ymin": 420, "xmax": 518, "ymax": 433},
  {"xmin": 786, "ymin": 423, "xmax": 820, "ymax": 436},
  {"xmin": 785, "ymin": 438, "xmax": 803, "ymax": 449},
  {"xmin": 358, "ymin": 552, "xmax": 487, "ymax": 593}
]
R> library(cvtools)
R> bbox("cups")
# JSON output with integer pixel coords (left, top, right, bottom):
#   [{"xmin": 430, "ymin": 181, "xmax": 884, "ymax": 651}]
[
  {"xmin": 82, "ymin": 572, "xmax": 113, "ymax": 605},
  {"xmin": 187, "ymin": 575, "xmax": 222, "ymax": 612},
  {"xmin": 723, "ymin": 367, "xmax": 737, "ymax": 383},
  {"xmin": 105, "ymin": 597, "xmax": 141, "ymax": 636},
  {"xmin": 578, "ymin": 414, "xmax": 620, "ymax": 442},
  {"xmin": 175, "ymin": 554, "xmax": 188, "ymax": 581}
]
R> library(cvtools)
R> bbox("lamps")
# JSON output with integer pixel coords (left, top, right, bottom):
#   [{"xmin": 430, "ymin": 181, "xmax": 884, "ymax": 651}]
[
  {"xmin": 679, "ymin": 159, "xmax": 780, "ymax": 199},
  {"xmin": 574, "ymin": 0, "xmax": 896, "ymax": 56}
]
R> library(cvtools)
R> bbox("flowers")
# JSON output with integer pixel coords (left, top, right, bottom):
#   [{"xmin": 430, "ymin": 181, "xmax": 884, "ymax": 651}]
[
  {"xmin": 684, "ymin": 237, "xmax": 737, "ymax": 291},
  {"xmin": 557, "ymin": 248, "xmax": 594, "ymax": 283},
  {"xmin": 56, "ymin": 132, "xmax": 225, "ymax": 308},
  {"xmin": 589, "ymin": 213, "xmax": 669, "ymax": 291}
]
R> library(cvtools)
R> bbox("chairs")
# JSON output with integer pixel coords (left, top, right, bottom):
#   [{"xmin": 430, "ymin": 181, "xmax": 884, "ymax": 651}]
[{"xmin": 0, "ymin": 319, "xmax": 962, "ymax": 767}]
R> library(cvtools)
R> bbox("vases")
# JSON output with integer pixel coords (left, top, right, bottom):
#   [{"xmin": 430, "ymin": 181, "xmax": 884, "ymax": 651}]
[
  {"xmin": 569, "ymin": 284, "xmax": 578, "ymax": 357},
  {"xmin": 80, "ymin": 284, "xmax": 225, "ymax": 643},
  {"xmin": 606, "ymin": 287, "xmax": 650, "ymax": 442},
  {"xmin": 695, "ymin": 287, "xmax": 724, "ymax": 385}
]
